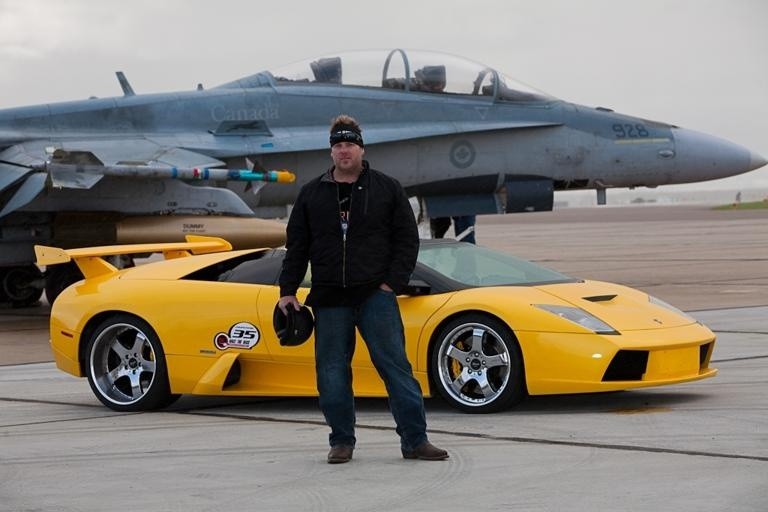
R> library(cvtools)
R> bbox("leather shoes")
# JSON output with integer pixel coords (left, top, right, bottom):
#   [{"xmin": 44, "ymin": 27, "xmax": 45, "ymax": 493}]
[
  {"xmin": 401, "ymin": 440, "xmax": 449, "ymax": 460},
  {"xmin": 327, "ymin": 445, "xmax": 354, "ymax": 463}
]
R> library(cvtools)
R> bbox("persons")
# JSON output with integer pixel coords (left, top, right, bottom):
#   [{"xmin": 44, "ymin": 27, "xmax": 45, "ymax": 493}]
[{"xmin": 278, "ymin": 114, "xmax": 450, "ymax": 463}]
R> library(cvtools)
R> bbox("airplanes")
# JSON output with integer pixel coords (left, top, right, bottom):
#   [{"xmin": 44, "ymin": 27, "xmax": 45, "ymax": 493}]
[{"xmin": 2, "ymin": 48, "xmax": 768, "ymax": 318}]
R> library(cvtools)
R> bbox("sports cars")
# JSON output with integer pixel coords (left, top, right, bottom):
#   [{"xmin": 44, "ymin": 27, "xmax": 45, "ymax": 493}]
[{"xmin": 33, "ymin": 226, "xmax": 716, "ymax": 416}]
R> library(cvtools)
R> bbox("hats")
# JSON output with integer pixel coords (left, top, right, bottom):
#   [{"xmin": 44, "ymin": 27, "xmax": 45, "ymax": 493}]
[{"xmin": 273, "ymin": 301, "xmax": 312, "ymax": 345}]
